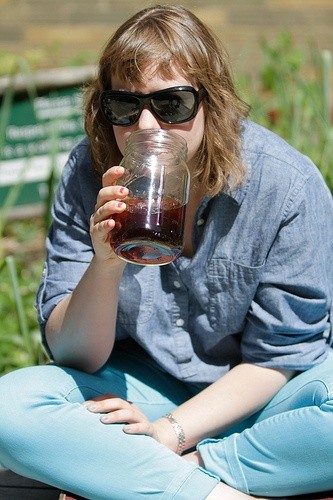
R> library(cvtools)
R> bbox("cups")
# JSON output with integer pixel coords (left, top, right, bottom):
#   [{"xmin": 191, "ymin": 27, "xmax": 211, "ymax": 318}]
[{"xmin": 110, "ymin": 129, "xmax": 189, "ymax": 267}]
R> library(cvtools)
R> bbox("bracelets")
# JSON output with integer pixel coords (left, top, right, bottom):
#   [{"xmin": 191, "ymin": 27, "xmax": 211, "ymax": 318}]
[{"xmin": 164, "ymin": 412, "xmax": 186, "ymax": 455}]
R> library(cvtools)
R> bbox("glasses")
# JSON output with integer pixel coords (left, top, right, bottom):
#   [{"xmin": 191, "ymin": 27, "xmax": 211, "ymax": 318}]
[{"xmin": 100, "ymin": 86, "xmax": 208, "ymax": 127}]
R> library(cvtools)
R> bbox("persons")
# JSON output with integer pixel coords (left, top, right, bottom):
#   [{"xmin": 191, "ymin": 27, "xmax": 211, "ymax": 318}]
[{"xmin": 0, "ymin": 6, "xmax": 333, "ymax": 500}]
